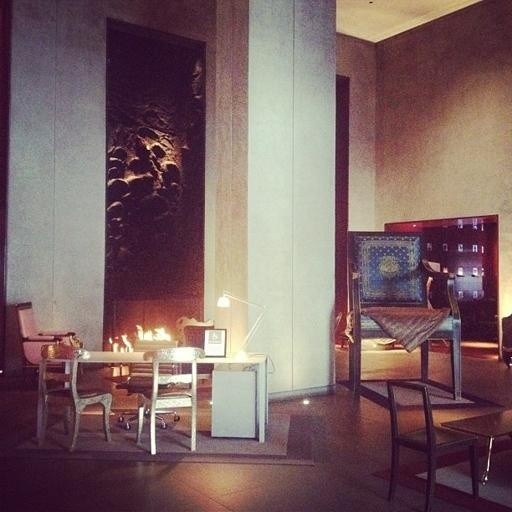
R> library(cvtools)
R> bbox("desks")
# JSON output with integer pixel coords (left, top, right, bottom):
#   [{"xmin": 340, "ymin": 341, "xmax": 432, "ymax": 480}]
[
  {"xmin": 35, "ymin": 350, "xmax": 271, "ymax": 442},
  {"xmin": 443, "ymin": 407, "xmax": 512, "ymax": 485}
]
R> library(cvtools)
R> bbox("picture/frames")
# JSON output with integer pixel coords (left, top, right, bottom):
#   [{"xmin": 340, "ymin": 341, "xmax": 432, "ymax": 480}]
[{"xmin": 204, "ymin": 329, "xmax": 227, "ymax": 358}]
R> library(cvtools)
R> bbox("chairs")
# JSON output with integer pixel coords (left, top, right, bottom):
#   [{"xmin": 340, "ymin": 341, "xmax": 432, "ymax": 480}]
[
  {"xmin": 41, "ymin": 358, "xmax": 112, "ymax": 451},
  {"xmin": 136, "ymin": 345, "xmax": 205, "ymax": 455},
  {"xmin": 389, "ymin": 382, "xmax": 478, "ymax": 510},
  {"xmin": 115, "ymin": 337, "xmax": 180, "ymax": 428},
  {"xmin": 18, "ymin": 301, "xmax": 83, "ymax": 381},
  {"xmin": 344, "ymin": 232, "xmax": 462, "ymax": 410}
]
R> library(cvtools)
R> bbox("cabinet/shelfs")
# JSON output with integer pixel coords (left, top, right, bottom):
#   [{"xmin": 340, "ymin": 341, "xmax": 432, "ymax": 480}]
[{"xmin": 211, "ymin": 367, "xmax": 264, "ymax": 439}]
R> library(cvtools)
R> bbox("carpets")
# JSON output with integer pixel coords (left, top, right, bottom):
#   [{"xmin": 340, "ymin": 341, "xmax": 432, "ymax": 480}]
[
  {"xmin": 12, "ymin": 408, "xmax": 315, "ymax": 468},
  {"xmin": 337, "ymin": 377, "xmax": 501, "ymax": 410},
  {"xmin": 377, "ymin": 437, "xmax": 512, "ymax": 512}
]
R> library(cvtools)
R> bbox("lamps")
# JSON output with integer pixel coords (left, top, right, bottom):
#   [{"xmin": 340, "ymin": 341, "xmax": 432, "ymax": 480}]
[{"xmin": 215, "ymin": 292, "xmax": 266, "ymax": 358}]
